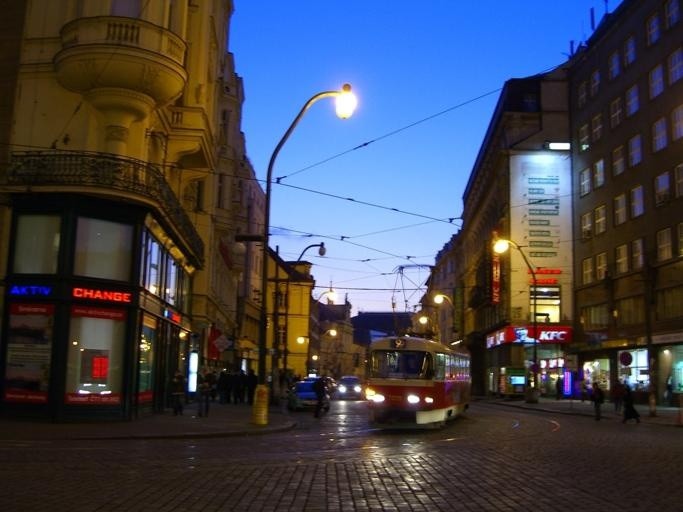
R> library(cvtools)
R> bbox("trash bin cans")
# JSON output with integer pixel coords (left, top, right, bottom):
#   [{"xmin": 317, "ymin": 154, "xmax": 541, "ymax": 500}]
[{"xmin": 524, "ymin": 388, "xmax": 538, "ymax": 403}]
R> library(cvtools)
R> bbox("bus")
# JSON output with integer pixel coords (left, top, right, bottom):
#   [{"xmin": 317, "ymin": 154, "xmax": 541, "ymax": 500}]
[{"xmin": 366, "ymin": 336, "xmax": 472, "ymax": 431}]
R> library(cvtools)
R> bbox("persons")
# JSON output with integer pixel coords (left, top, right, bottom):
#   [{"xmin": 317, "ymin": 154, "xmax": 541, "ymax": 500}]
[
  {"xmin": 311, "ymin": 374, "xmax": 328, "ymax": 418},
  {"xmin": 170, "ymin": 368, "xmax": 187, "ymax": 416},
  {"xmin": 613, "ymin": 379, "xmax": 623, "ymax": 410},
  {"xmin": 512, "ymin": 327, "xmax": 542, "ymax": 342},
  {"xmin": 591, "ymin": 382, "xmax": 603, "ymax": 420},
  {"xmin": 194, "ymin": 365, "xmax": 211, "ymax": 418},
  {"xmin": 620, "ymin": 386, "xmax": 641, "ymax": 423},
  {"xmin": 214, "ymin": 366, "xmax": 257, "ymax": 405},
  {"xmin": 554, "ymin": 376, "xmax": 564, "ymax": 401}
]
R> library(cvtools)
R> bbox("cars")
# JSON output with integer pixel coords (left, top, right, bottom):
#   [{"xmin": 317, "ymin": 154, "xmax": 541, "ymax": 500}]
[{"xmin": 295, "ymin": 374, "xmax": 363, "ymax": 410}]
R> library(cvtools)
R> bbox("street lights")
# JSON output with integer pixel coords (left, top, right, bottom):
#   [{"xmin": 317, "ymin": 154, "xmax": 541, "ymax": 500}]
[
  {"xmin": 493, "ymin": 239, "xmax": 538, "ymax": 388},
  {"xmin": 311, "ymin": 289, "xmax": 335, "ymax": 312},
  {"xmin": 418, "ymin": 316, "xmax": 433, "ymax": 326},
  {"xmin": 250, "ymin": 83, "xmax": 358, "ymax": 425},
  {"xmin": 283, "ymin": 242, "xmax": 326, "ymax": 371},
  {"xmin": 318, "ymin": 329, "xmax": 337, "ymax": 347},
  {"xmin": 433, "ymin": 294, "xmax": 455, "ymax": 311}
]
